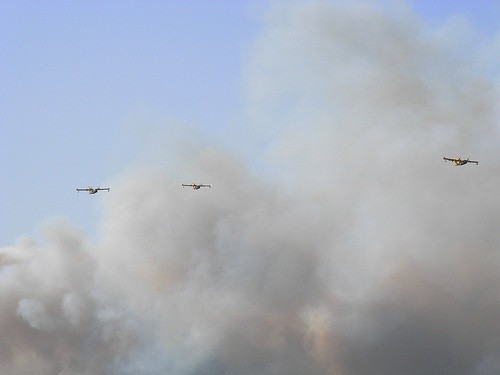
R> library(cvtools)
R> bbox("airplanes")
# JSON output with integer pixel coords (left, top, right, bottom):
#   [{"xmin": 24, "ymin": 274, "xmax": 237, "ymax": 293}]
[
  {"xmin": 182, "ymin": 184, "xmax": 210, "ymax": 190},
  {"xmin": 77, "ymin": 187, "xmax": 109, "ymax": 195},
  {"xmin": 443, "ymin": 156, "xmax": 479, "ymax": 167}
]
